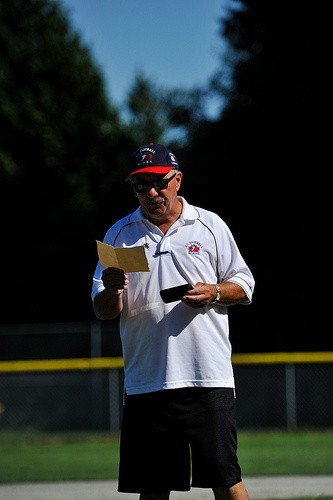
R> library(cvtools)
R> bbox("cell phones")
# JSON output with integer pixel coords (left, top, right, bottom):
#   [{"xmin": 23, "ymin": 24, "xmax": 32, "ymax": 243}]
[{"xmin": 160, "ymin": 284, "xmax": 194, "ymax": 303}]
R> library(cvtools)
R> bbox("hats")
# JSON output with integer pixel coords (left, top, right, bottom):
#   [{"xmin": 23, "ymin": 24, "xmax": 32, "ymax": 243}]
[{"xmin": 124, "ymin": 142, "xmax": 178, "ymax": 182}]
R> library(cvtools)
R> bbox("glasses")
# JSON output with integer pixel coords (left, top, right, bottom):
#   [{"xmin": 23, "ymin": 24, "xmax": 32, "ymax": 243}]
[{"xmin": 130, "ymin": 173, "xmax": 176, "ymax": 193}]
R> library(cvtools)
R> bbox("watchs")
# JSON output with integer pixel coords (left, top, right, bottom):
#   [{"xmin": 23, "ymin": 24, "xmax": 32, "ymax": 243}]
[{"xmin": 210, "ymin": 284, "xmax": 221, "ymax": 306}]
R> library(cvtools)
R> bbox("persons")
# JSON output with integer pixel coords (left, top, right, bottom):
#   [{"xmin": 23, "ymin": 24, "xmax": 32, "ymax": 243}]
[{"xmin": 91, "ymin": 143, "xmax": 255, "ymax": 500}]
